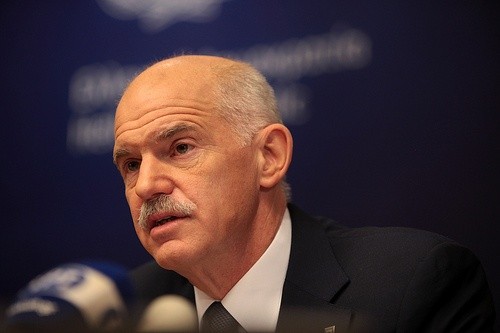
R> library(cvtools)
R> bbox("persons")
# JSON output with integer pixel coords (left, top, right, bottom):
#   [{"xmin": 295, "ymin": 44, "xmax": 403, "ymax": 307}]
[{"xmin": 109, "ymin": 51, "xmax": 497, "ymax": 333}]
